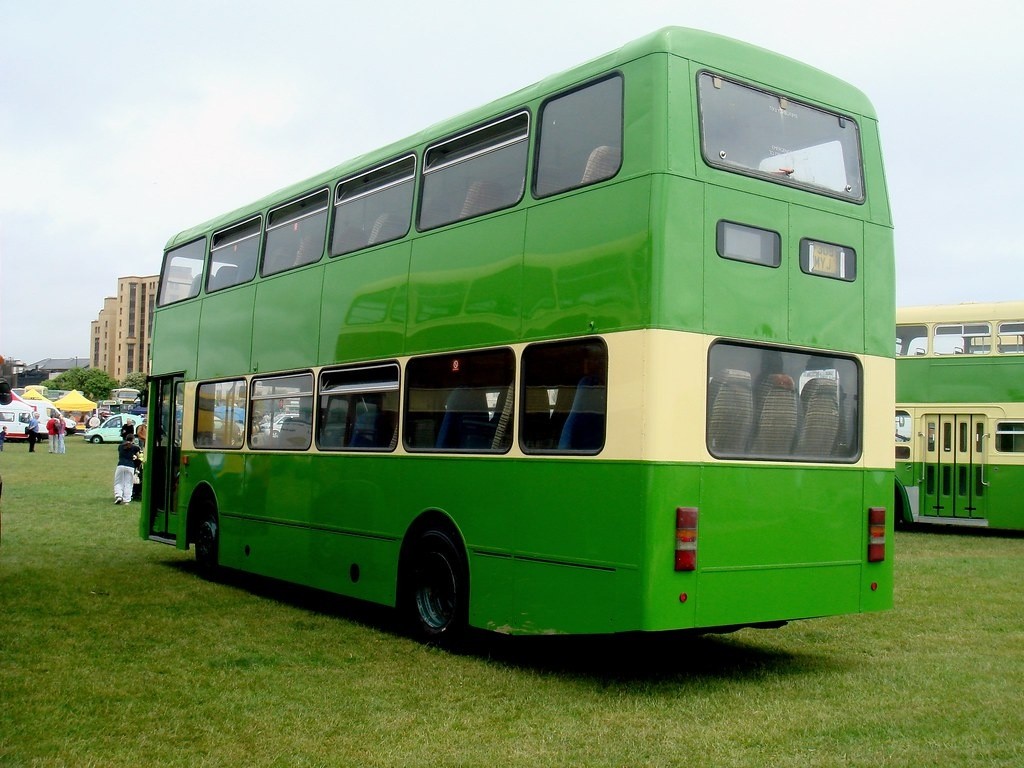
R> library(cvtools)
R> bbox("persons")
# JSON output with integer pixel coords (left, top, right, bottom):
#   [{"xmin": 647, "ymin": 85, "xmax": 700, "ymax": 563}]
[
  {"xmin": 137, "ymin": 419, "xmax": 147, "ymax": 449},
  {"xmin": 67, "ymin": 410, "xmax": 106, "ymax": 428},
  {"xmin": 28, "ymin": 413, "xmax": 40, "ymax": 452},
  {"xmin": 114, "ymin": 434, "xmax": 140, "ymax": 505},
  {"xmin": 121, "ymin": 419, "xmax": 136, "ymax": 444},
  {"xmin": 0, "ymin": 426, "xmax": 7, "ymax": 451},
  {"xmin": 46, "ymin": 414, "xmax": 66, "ymax": 453}
]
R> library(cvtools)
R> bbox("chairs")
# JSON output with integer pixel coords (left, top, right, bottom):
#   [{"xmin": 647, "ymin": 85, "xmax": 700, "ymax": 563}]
[
  {"xmin": 708, "ymin": 370, "xmax": 840, "ymax": 456},
  {"xmin": 517, "ymin": 161, "xmax": 579, "ymax": 203},
  {"xmin": 212, "ymin": 266, "xmax": 238, "ymax": 291},
  {"xmin": 954, "ymin": 346, "xmax": 964, "ymax": 354},
  {"xmin": 460, "ymin": 182, "xmax": 510, "ymax": 219},
  {"xmin": 367, "ymin": 211, "xmax": 410, "ymax": 245},
  {"xmin": 350, "ymin": 378, "xmax": 608, "ymax": 450},
  {"xmin": 579, "ymin": 145, "xmax": 621, "ymax": 185},
  {"xmin": 294, "ymin": 231, "xmax": 324, "ymax": 268},
  {"xmin": 235, "ymin": 256, "xmax": 256, "ymax": 284},
  {"xmin": 188, "ymin": 273, "xmax": 215, "ymax": 297},
  {"xmin": 914, "ymin": 347, "xmax": 925, "ymax": 355}
]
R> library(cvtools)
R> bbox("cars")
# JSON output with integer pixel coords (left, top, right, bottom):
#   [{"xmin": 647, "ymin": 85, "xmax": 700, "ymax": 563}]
[{"xmin": 0, "ymin": 381, "xmax": 300, "ymax": 446}]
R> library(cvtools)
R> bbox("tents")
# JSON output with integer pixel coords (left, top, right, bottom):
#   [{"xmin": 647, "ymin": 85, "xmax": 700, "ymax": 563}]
[
  {"xmin": 54, "ymin": 388, "xmax": 98, "ymax": 424},
  {"xmin": 20, "ymin": 389, "xmax": 53, "ymax": 403}
]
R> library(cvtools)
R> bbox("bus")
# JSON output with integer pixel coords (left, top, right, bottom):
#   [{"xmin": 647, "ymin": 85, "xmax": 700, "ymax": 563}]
[
  {"xmin": 135, "ymin": 24, "xmax": 899, "ymax": 652},
  {"xmin": 895, "ymin": 299, "xmax": 1024, "ymax": 536}
]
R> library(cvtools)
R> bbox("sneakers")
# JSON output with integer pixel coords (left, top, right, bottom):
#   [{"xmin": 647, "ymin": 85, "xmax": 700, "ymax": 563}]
[{"xmin": 114, "ymin": 497, "xmax": 123, "ymax": 505}]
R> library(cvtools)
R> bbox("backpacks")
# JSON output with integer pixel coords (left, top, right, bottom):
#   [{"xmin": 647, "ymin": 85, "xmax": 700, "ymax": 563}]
[{"xmin": 53, "ymin": 419, "xmax": 60, "ymax": 433}]
